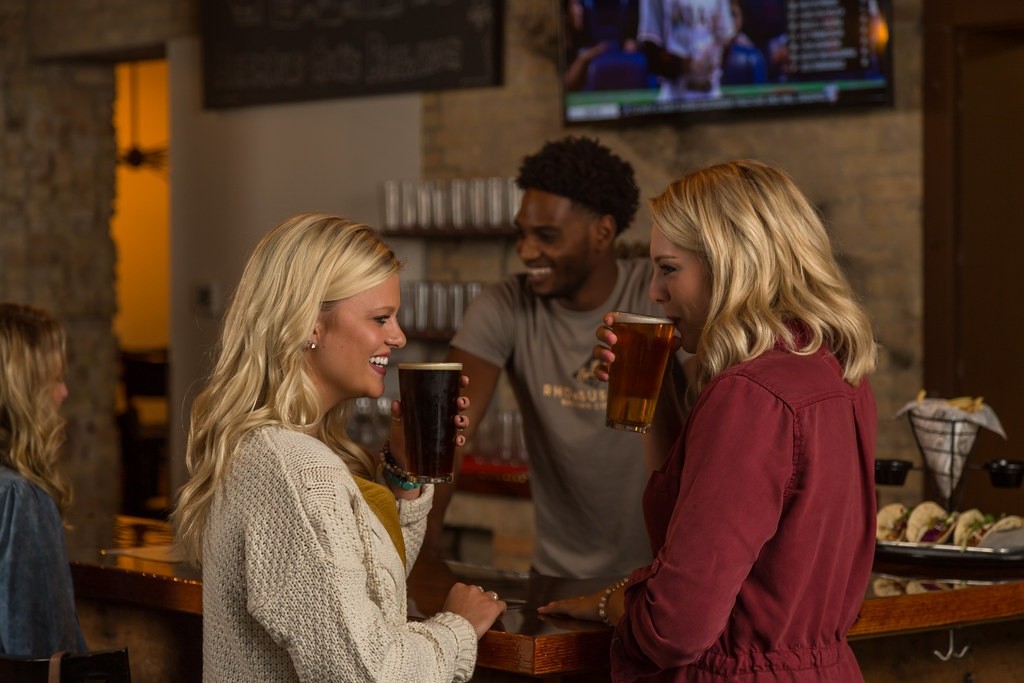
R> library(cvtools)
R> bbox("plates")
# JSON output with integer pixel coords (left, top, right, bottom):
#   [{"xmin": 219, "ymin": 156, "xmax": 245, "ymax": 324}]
[
  {"xmin": 876, "ymin": 539, "xmax": 1024, "ymax": 554},
  {"xmin": 442, "ymin": 558, "xmax": 531, "ymax": 583}
]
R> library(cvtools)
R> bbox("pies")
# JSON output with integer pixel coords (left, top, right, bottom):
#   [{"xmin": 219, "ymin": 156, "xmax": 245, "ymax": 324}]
[{"xmin": 874, "ymin": 500, "xmax": 1024, "ymax": 597}]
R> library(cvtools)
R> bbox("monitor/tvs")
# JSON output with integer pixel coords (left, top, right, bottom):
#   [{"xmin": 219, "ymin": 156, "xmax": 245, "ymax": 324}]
[{"xmin": 559, "ymin": 0, "xmax": 895, "ymax": 130}]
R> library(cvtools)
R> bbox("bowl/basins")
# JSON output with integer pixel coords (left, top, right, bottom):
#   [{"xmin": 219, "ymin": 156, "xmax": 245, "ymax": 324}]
[
  {"xmin": 987, "ymin": 461, "xmax": 1024, "ymax": 487},
  {"xmin": 874, "ymin": 459, "xmax": 911, "ymax": 486}
]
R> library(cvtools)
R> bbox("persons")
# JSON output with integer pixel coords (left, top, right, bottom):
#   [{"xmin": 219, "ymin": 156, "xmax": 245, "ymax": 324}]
[
  {"xmin": 537, "ymin": 159, "xmax": 877, "ymax": 683},
  {"xmin": 406, "ymin": 137, "xmax": 702, "ymax": 619},
  {"xmin": 174, "ymin": 212, "xmax": 503, "ymax": 683},
  {"xmin": 0, "ymin": 304, "xmax": 93, "ymax": 660}
]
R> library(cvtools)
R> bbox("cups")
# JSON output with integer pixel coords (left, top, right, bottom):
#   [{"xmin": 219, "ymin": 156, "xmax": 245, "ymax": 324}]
[
  {"xmin": 392, "ymin": 281, "xmax": 481, "ymax": 332},
  {"xmin": 603, "ymin": 312, "xmax": 674, "ymax": 432},
  {"xmin": 358, "ymin": 394, "xmax": 392, "ymax": 444},
  {"xmin": 384, "ymin": 173, "xmax": 524, "ymax": 229},
  {"xmin": 398, "ymin": 364, "xmax": 465, "ymax": 483}
]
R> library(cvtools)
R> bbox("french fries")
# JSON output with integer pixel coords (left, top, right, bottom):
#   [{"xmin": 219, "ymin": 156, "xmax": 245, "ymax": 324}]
[{"xmin": 915, "ymin": 390, "xmax": 983, "ymax": 413}]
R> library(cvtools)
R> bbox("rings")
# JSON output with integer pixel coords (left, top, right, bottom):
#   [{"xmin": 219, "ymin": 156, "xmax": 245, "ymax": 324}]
[
  {"xmin": 488, "ymin": 591, "xmax": 498, "ymax": 600},
  {"xmin": 477, "ymin": 585, "xmax": 484, "ymax": 592},
  {"xmin": 578, "ymin": 594, "xmax": 585, "ymax": 601},
  {"xmin": 390, "ymin": 414, "xmax": 403, "ymax": 422}
]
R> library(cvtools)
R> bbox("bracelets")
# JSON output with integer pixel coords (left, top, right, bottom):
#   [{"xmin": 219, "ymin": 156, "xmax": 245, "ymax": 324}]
[
  {"xmin": 380, "ymin": 439, "xmax": 423, "ymax": 491},
  {"xmin": 598, "ymin": 576, "xmax": 631, "ymax": 627}
]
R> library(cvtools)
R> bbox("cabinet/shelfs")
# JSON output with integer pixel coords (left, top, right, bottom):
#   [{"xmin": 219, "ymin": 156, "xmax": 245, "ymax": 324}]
[{"xmin": 370, "ymin": 217, "xmax": 528, "ymax": 498}]
